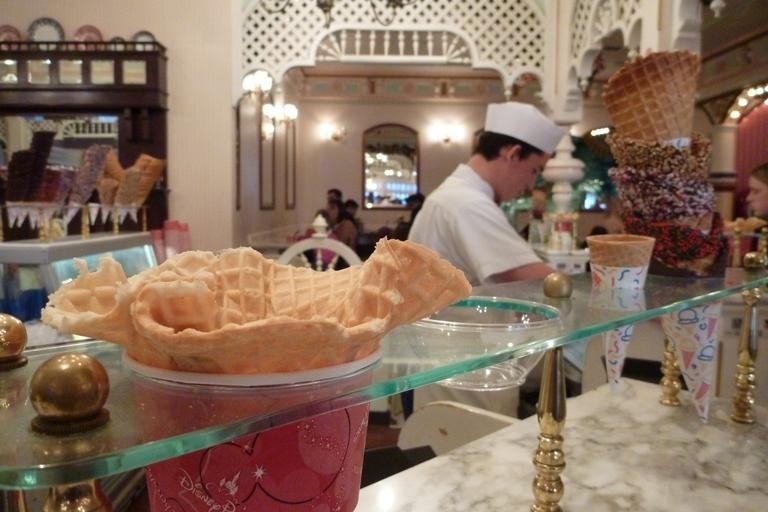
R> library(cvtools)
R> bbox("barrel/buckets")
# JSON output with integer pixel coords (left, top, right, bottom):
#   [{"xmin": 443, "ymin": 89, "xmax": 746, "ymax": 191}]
[{"xmin": 126, "ymin": 371, "xmax": 372, "ymax": 512}]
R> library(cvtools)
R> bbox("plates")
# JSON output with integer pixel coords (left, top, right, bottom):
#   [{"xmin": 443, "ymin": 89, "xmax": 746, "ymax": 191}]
[{"xmin": 0, "ymin": 18, "xmax": 157, "ymax": 53}]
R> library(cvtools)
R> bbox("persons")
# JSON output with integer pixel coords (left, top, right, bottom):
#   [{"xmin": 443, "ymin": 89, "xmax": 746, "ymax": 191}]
[
  {"xmin": 395, "ymin": 192, "xmax": 425, "ymax": 241},
  {"xmin": 327, "ymin": 188, "xmax": 342, "ymax": 199},
  {"xmin": 327, "ymin": 199, "xmax": 358, "ymax": 255},
  {"xmin": 399, "ymin": 102, "xmax": 568, "ymax": 466},
  {"xmin": 345, "ymin": 200, "xmax": 358, "ymax": 215},
  {"xmin": 746, "ymin": 162, "xmax": 767, "ymax": 253},
  {"xmin": 287, "ymin": 208, "xmax": 337, "ymax": 270}
]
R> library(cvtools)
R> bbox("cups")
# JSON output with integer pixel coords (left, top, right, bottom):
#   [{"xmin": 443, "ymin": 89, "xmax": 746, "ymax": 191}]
[{"xmin": 121, "ymin": 332, "xmax": 381, "ymax": 511}]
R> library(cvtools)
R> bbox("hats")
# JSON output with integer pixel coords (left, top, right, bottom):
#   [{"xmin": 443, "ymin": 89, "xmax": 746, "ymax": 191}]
[{"xmin": 484, "ymin": 98, "xmax": 567, "ymax": 158}]
